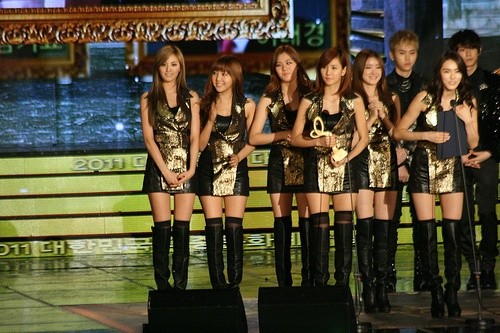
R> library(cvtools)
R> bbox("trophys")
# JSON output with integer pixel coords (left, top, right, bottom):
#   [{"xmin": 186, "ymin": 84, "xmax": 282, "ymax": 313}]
[{"xmin": 309, "ymin": 115, "xmax": 348, "ymax": 162}]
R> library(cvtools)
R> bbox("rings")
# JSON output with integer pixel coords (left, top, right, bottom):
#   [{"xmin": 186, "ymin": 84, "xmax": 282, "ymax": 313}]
[{"xmin": 474, "ymin": 161, "xmax": 478, "ymax": 165}]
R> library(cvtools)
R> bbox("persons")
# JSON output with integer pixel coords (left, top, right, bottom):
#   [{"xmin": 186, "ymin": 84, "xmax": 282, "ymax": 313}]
[
  {"xmin": 291, "ymin": 47, "xmax": 368, "ymax": 285},
  {"xmin": 138, "ymin": 45, "xmax": 201, "ymax": 290},
  {"xmin": 201, "ymin": 56, "xmax": 256, "ymax": 290},
  {"xmin": 447, "ymin": 30, "xmax": 499, "ymax": 290},
  {"xmin": 378, "ymin": 30, "xmax": 431, "ymax": 293},
  {"xmin": 247, "ymin": 44, "xmax": 315, "ymax": 287},
  {"xmin": 349, "ymin": 50, "xmax": 401, "ymax": 315},
  {"xmin": 392, "ymin": 52, "xmax": 479, "ymax": 320}
]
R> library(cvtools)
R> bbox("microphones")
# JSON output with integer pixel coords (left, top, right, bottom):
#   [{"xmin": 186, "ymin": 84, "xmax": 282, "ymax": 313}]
[
  {"xmin": 450, "ymin": 100, "xmax": 482, "ymax": 302},
  {"xmin": 340, "ymin": 101, "xmax": 362, "ymax": 285}
]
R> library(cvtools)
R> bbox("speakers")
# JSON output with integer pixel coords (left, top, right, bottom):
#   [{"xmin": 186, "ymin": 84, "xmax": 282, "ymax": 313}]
[
  {"xmin": 257, "ymin": 284, "xmax": 359, "ymax": 333},
  {"xmin": 147, "ymin": 289, "xmax": 249, "ymax": 333}
]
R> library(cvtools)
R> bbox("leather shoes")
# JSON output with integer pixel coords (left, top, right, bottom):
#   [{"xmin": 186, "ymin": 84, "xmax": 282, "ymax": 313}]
[
  {"xmin": 466, "ymin": 275, "xmax": 478, "ymax": 292},
  {"xmin": 480, "ymin": 270, "xmax": 496, "ymax": 288}
]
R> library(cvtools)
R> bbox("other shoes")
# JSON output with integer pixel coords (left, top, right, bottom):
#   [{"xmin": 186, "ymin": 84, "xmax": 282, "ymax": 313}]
[
  {"xmin": 413, "ymin": 278, "xmax": 434, "ymax": 291},
  {"xmin": 387, "ymin": 277, "xmax": 396, "ymax": 292}
]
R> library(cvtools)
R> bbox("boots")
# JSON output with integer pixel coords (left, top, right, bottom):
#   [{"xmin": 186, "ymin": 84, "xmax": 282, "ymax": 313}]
[
  {"xmin": 274, "ymin": 220, "xmax": 293, "ymax": 288},
  {"xmin": 308, "ymin": 227, "xmax": 330, "ymax": 287},
  {"xmin": 205, "ymin": 226, "xmax": 227, "ymax": 289},
  {"xmin": 299, "ymin": 221, "xmax": 315, "ymax": 287},
  {"xmin": 416, "ymin": 219, "xmax": 445, "ymax": 317},
  {"xmin": 355, "ymin": 217, "xmax": 380, "ymax": 315},
  {"xmin": 372, "ymin": 219, "xmax": 391, "ymax": 313},
  {"xmin": 440, "ymin": 218, "xmax": 463, "ymax": 317},
  {"xmin": 224, "ymin": 226, "xmax": 245, "ymax": 289},
  {"xmin": 334, "ymin": 223, "xmax": 353, "ymax": 287},
  {"xmin": 172, "ymin": 227, "xmax": 190, "ymax": 290},
  {"xmin": 151, "ymin": 226, "xmax": 172, "ymax": 289}
]
